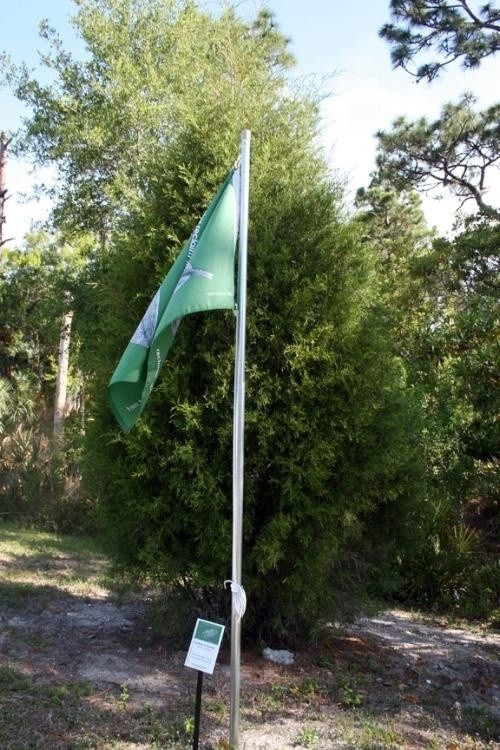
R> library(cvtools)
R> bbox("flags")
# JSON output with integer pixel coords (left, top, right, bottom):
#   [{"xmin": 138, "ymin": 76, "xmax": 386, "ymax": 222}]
[{"xmin": 107, "ymin": 157, "xmax": 240, "ymax": 436}]
